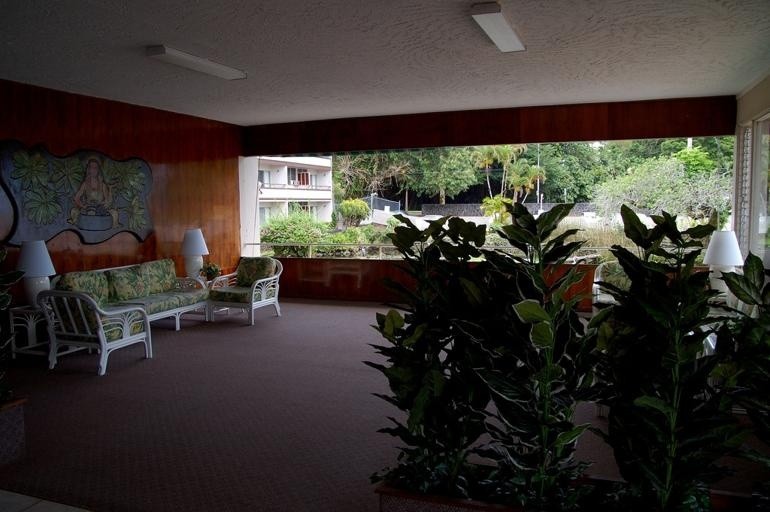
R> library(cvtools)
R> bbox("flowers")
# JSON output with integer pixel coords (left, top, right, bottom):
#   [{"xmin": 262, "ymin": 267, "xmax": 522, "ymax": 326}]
[{"xmin": 200, "ymin": 261, "xmax": 224, "ymax": 276}]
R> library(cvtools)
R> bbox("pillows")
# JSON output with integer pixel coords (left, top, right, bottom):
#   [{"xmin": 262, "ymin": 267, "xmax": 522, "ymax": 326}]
[
  {"xmin": 237, "ymin": 257, "xmax": 277, "ymax": 287},
  {"xmin": 56, "ymin": 258, "xmax": 177, "ymax": 327}
]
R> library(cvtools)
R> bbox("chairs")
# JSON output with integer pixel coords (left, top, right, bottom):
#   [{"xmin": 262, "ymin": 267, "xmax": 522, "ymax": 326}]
[
  {"xmin": 210, "ymin": 257, "xmax": 284, "ymax": 325},
  {"xmin": 591, "ymin": 261, "xmax": 632, "ymax": 319},
  {"xmin": 37, "ymin": 290, "xmax": 153, "ymax": 376}
]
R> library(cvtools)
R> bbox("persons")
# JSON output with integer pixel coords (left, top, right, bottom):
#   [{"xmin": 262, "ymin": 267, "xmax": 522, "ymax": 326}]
[{"xmin": 68, "ymin": 154, "xmax": 121, "ymax": 230}]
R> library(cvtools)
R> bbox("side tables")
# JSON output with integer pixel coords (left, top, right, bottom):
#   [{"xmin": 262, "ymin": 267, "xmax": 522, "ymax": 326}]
[{"xmin": 10, "ymin": 305, "xmax": 92, "ymax": 359}]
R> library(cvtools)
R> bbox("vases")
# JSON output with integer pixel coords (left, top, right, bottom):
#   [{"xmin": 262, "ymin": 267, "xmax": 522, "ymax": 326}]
[{"xmin": 207, "ymin": 272, "xmax": 217, "ymax": 281}]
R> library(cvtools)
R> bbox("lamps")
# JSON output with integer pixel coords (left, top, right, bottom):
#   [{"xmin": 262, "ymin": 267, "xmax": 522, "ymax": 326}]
[
  {"xmin": 146, "ymin": 44, "xmax": 247, "ymax": 81},
  {"xmin": 179, "ymin": 228, "xmax": 209, "ymax": 277},
  {"xmin": 16, "ymin": 239, "xmax": 56, "ymax": 309},
  {"xmin": 702, "ymin": 229, "xmax": 745, "ymax": 293},
  {"xmin": 470, "ymin": 2, "xmax": 526, "ymax": 53}
]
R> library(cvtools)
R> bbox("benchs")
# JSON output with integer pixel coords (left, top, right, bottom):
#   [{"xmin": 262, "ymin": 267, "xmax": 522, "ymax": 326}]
[{"xmin": 50, "ymin": 258, "xmax": 210, "ymax": 354}]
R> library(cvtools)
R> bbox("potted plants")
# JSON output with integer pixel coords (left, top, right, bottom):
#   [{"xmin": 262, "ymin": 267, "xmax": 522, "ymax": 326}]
[{"xmin": 361, "ymin": 200, "xmax": 770, "ymax": 511}]
